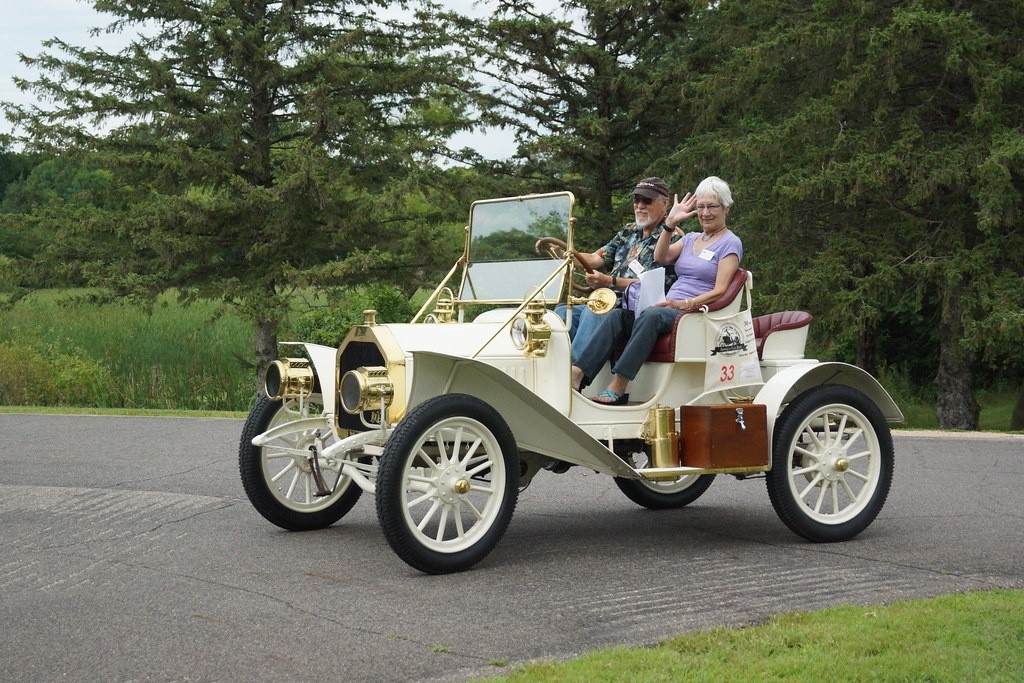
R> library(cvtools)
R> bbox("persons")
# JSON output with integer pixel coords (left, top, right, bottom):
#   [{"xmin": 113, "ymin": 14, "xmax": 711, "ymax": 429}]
[
  {"xmin": 572, "ymin": 176, "xmax": 743, "ymax": 405},
  {"xmin": 535, "ymin": 177, "xmax": 682, "ymax": 366}
]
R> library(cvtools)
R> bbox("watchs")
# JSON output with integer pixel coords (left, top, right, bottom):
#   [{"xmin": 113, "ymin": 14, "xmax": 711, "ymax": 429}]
[{"xmin": 609, "ymin": 276, "xmax": 616, "ymax": 290}]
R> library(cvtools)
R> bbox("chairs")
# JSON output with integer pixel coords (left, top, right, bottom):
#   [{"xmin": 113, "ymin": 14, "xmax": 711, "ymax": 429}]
[{"xmin": 753, "ymin": 310, "xmax": 813, "ymax": 358}]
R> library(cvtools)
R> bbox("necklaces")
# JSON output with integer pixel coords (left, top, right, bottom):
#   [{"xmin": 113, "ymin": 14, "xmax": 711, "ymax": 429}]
[{"xmin": 702, "ymin": 226, "xmax": 726, "ymax": 241}]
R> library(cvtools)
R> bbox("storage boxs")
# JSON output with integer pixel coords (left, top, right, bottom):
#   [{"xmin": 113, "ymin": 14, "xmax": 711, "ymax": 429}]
[{"xmin": 679, "ymin": 403, "xmax": 768, "ymax": 469}]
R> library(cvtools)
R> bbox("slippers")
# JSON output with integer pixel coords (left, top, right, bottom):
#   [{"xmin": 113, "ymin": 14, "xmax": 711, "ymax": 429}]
[{"xmin": 591, "ymin": 387, "xmax": 630, "ymax": 405}]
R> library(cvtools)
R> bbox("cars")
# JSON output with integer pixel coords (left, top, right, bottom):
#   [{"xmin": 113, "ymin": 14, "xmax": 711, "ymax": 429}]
[{"xmin": 237, "ymin": 190, "xmax": 905, "ymax": 575}]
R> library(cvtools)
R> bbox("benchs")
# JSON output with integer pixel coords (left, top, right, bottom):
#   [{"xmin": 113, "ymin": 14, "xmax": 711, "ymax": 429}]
[{"xmin": 615, "ymin": 267, "xmax": 750, "ymax": 363}]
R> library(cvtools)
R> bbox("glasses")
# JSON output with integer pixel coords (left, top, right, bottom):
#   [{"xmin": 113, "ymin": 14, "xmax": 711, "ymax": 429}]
[
  {"xmin": 695, "ymin": 203, "xmax": 722, "ymax": 212},
  {"xmin": 633, "ymin": 197, "xmax": 657, "ymax": 205}
]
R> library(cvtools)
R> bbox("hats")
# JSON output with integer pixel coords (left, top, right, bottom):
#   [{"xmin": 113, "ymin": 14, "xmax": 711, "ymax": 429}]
[{"xmin": 630, "ymin": 176, "xmax": 669, "ymax": 199}]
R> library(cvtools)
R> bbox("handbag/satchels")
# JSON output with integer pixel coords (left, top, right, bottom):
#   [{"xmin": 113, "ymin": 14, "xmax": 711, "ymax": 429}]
[{"xmin": 622, "ymin": 280, "xmax": 642, "ymax": 338}]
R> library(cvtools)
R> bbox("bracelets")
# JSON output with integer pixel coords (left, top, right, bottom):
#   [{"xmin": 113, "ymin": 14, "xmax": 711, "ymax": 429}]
[
  {"xmin": 691, "ymin": 298, "xmax": 696, "ymax": 307},
  {"xmin": 662, "ymin": 221, "xmax": 676, "ymax": 233},
  {"xmin": 685, "ymin": 298, "xmax": 689, "ymax": 308}
]
